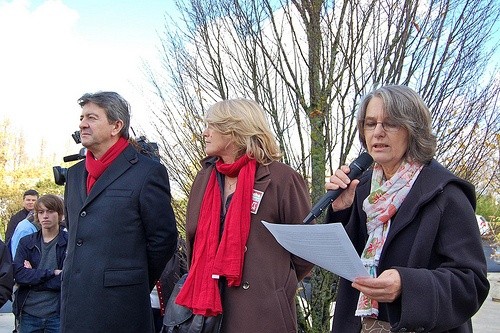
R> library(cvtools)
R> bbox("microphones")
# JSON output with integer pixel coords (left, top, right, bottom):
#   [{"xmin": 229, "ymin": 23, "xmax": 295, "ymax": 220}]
[
  {"xmin": 63, "ymin": 154, "xmax": 86, "ymax": 162},
  {"xmin": 303, "ymin": 152, "xmax": 375, "ymax": 224}
]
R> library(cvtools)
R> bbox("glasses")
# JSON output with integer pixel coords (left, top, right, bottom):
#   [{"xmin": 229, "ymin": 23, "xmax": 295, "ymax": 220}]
[{"xmin": 364, "ymin": 116, "xmax": 401, "ymax": 131}]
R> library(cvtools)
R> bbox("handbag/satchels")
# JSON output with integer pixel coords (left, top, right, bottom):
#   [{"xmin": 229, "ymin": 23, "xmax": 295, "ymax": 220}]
[{"xmin": 160, "ymin": 273, "xmax": 223, "ymax": 333}]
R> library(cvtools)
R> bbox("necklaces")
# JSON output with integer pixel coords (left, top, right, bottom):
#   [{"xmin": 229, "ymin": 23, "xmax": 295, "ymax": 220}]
[{"xmin": 226, "ymin": 175, "xmax": 237, "ymax": 190}]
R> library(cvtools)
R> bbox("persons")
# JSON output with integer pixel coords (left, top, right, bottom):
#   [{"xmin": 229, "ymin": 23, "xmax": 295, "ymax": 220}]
[
  {"xmin": 324, "ymin": 84, "xmax": 490, "ymax": 333},
  {"xmin": 13, "ymin": 194, "xmax": 69, "ymax": 333},
  {"xmin": 60, "ymin": 91, "xmax": 178, "ymax": 333},
  {"xmin": 150, "ymin": 254, "xmax": 189, "ymax": 333},
  {"xmin": 0, "ymin": 189, "xmax": 68, "ymax": 309},
  {"xmin": 174, "ymin": 98, "xmax": 316, "ymax": 333}
]
